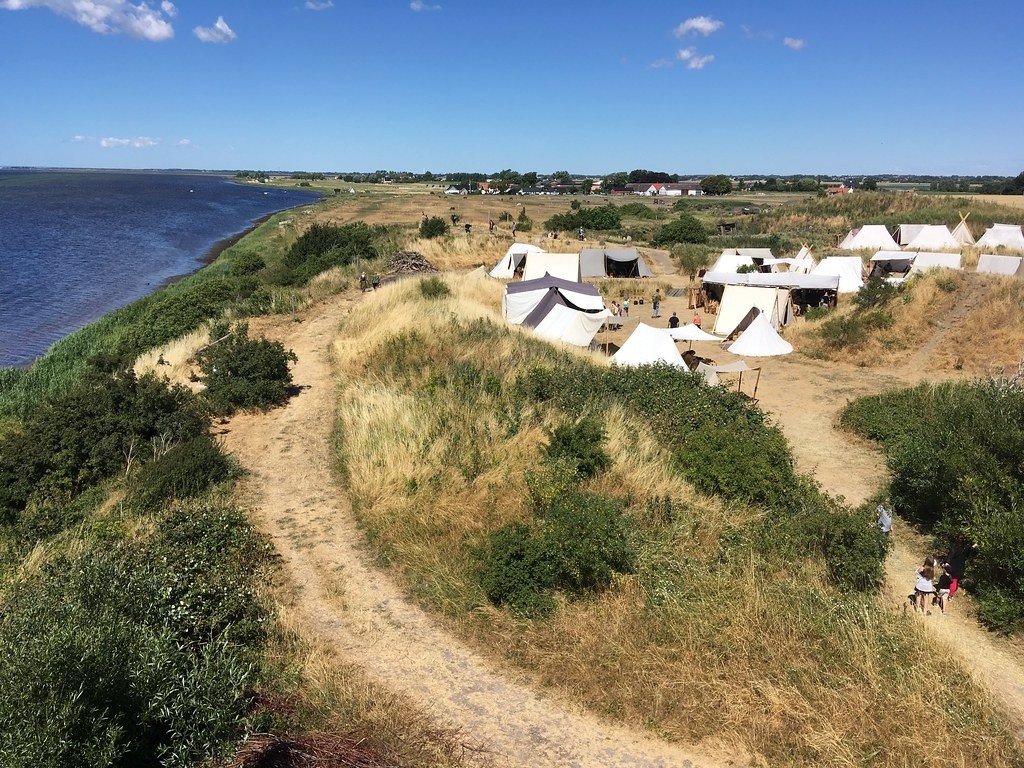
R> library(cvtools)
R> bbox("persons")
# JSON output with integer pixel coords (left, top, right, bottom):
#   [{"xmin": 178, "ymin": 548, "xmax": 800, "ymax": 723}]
[
  {"xmin": 616, "ymin": 304, "xmax": 622, "ymax": 316},
  {"xmin": 489, "ymin": 219, "xmax": 495, "ymax": 233},
  {"xmin": 611, "ymin": 300, "xmax": 620, "ymax": 317},
  {"xmin": 914, "ymin": 555, "xmax": 954, "ymax": 615},
  {"xmin": 554, "ymin": 232, "xmax": 558, "ymax": 239},
  {"xmin": 548, "ymin": 231, "xmax": 555, "ymax": 239},
  {"xmin": 651, "ymin": 288, "xmax": 662, "ymax": 318},
  {"xmin": 693, "ymin": 310, "xmax": 701, "ymax": 329},
  {"xmin": 874, "ymin": 498, "xmax": 894, "ymax": 539},
  {"xmin": 578, "ymin": 226, "xmax": 585, "ymax": 239},
  {"xmin": 418, "ymin": 213, "xmax": 472, "ymax": 233},
  {"xmin": 359, "ymin": 271, "xmax": 380, "ymax": 293},
  {"xmin": 622, "ymin": 297, "xmax": 629, "ymax": 317},
  {"xmin": 667, "ymin": 312, "xmax": 679, "ymax": 328},
  {"xmin": 821, "ymin": 292, "xmax": 829, "ymax": 311},
  {"xmin": 511, "ymin": 222, "xmax": 517, "ymax": 237},
  {"xmin": 681, "ymin": 349, "xmax": 696, "ymax": 367}
]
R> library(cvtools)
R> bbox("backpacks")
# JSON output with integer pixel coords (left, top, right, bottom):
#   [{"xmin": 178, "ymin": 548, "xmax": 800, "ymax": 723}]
[{"xmin": 652, "ymin": 294, "xmax": 659, "ymax": 303}]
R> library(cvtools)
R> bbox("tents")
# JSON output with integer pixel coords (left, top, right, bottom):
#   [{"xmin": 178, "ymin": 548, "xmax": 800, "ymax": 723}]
[{"xmin": 488, "ymin": 223, "xmax": 1024, "ymax": 388}]
